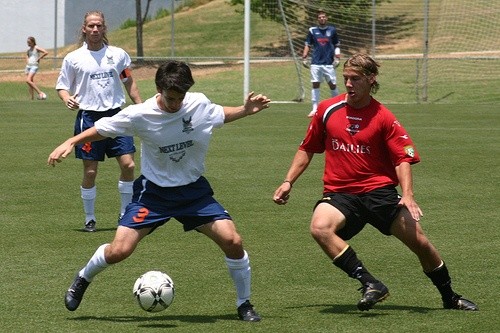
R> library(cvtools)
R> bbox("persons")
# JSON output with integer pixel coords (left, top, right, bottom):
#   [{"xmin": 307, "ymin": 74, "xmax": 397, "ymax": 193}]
[
  {"xmin": 273, "ymin": 54, "xmax": 480, "ymax": 311},
  {"xmin": 25, "ymin": 37, "xmax": 48, "ymax": 100},
  {"xmin": 46, "ymin": 61, "xmax": 271, "ymax": 321},
  {"xmin": 301, "ymin": 11, "xmax": 341, "ymax": 118},
  {"xmin": 53, "ymin": 11, "xmax": 143, "ymax": 231}
]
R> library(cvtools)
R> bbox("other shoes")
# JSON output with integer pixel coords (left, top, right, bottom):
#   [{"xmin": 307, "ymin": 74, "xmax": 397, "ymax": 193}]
[{"xmin": 308, "ymin": 110, "xmax": 317, "ymax": 118}]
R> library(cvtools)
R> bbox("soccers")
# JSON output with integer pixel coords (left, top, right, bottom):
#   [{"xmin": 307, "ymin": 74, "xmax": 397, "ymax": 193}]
[{"xmin": 132, "ymin": 270, "xmax": 175, "ymax": 313}]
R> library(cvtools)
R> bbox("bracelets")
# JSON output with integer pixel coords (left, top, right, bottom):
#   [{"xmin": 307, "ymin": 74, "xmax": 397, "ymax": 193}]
[{"xmin": 284, "ymin": 181, "xmax": 293, "ymax": 187}]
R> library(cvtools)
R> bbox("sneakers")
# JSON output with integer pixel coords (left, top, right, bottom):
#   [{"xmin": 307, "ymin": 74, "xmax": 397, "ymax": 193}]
[
  {"xmin": 442, "ymin": 293, "xmax": 478, "ymax": 311},
  {"xmin": 83, "ymin": 219, "xmax": 96, "ymax": 232},
  {"xmin": 358, "ymin": 280, "xmax": 390, "ymax": 311},
  {"xmin": 237, "ymin": 300, "xmax": 260, "ymax": 322},
  {"xmin": 65, "ymin": 272, "xmax": 91, "ymax": 311}
]
